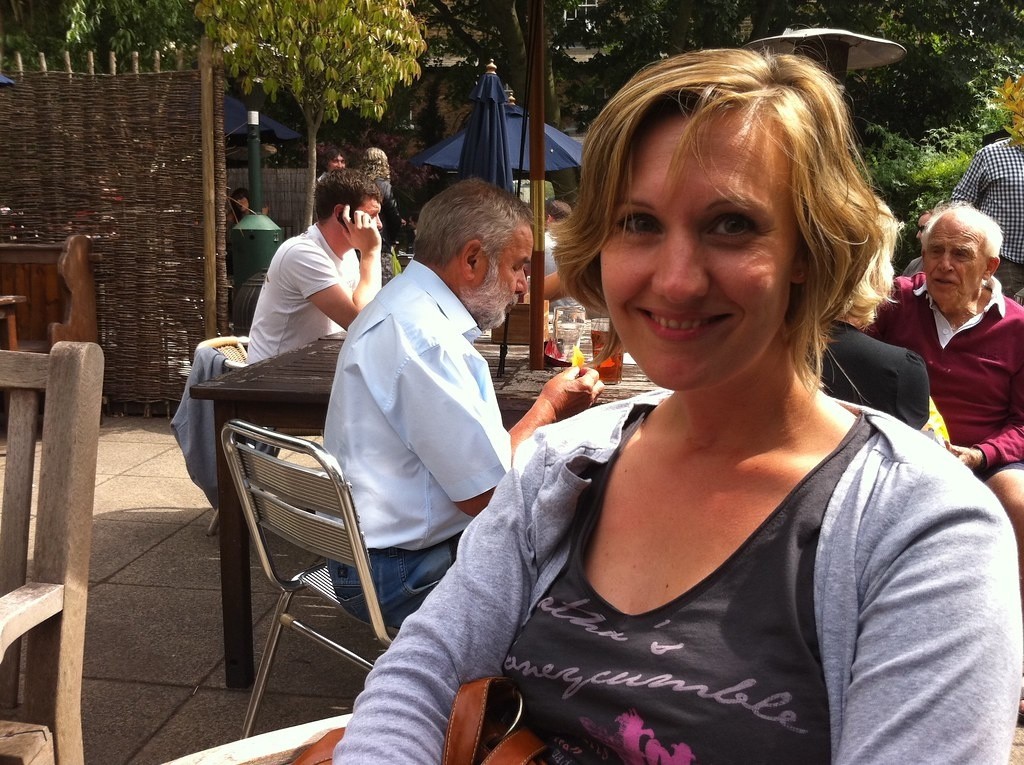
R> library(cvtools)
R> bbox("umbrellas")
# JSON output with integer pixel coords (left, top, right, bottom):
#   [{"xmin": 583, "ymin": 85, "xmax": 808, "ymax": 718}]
[
  {"xmin": 456, "ymin": 58, "xmax": 515, "ymax": 194},
  {"xmin": 409, "ymin": 93, "xmax": 582, "ymax": 171},
  {"xmin": 223, "ymin": 95, "xmax": 302, "ymax": 145}
]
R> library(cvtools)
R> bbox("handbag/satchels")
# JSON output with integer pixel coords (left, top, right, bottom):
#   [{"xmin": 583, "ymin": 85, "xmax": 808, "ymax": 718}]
[{"xmin": 286, "ymin": 677, "xmax": 547, "ymax": 765}]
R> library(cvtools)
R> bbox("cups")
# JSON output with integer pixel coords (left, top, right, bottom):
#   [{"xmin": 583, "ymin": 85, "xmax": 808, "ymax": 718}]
[
  {"xmin": 553, "ymin": 305, "xmax": 586, "ymax": 359},
  {"xmin": 381, "ymin": 253, "xmax": 394, "ymax": 282},
  {"xmin": 591, "ymin": 317, "xmax": 624, "ymax": 385}
]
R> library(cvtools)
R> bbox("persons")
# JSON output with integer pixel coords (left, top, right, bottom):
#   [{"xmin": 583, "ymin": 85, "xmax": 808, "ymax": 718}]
[
  {"xmin": 333, "ymin": 49, "xmax": 1023, "ymax": 765},
  {"xmin": 316, "ymin": 148, "xmax": 345, "ymax": 183},
  {"xmin": 862, "ymin": 207, "xmax": 1024, "ymax": 600},
  {"xmin": 359, "ymin": 148, "xmax": 406, "ymax": 288},
  {"xmin": 950, "ymin": 137, "xmax": 1024, "ymax": 300},
  {"xmin": 902, "ymin": 210, "xmax": 933, "ymax": 276},
  {"xmin": 545, "ymin": 199, "xmax": 586, "ymax": 313},
  {"xmin": 805, "ymin": 196, "xmax": 930, "ymax": 432},
  {"xmin": 246, "ymin": 167, "xmax": 385, "ymax": 366},
  {"xmin": 226, "ymin": 188, "xmax": 269, "ymax": 265},
  {"xmin": 316, "ymin": 178, "xmax": 605, "ymax": 629}
]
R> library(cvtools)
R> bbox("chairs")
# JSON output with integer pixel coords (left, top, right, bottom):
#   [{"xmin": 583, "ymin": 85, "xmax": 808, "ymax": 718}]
[
  {"xmin": 196, "ymin": 337, "xmax": 325, "ymax": 536},
  {"xmin": 0, "ymin": 341, "xmax": 105, "ymax": 765},
  {"xmin": 217, "ymin": 417, "xmax": 406, "ymax": 741}
]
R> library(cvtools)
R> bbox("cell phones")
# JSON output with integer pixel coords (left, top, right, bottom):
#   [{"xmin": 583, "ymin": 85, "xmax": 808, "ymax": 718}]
[{"xmin": 337, "ymin": 210, "xmax": 356, "ymax": 230}]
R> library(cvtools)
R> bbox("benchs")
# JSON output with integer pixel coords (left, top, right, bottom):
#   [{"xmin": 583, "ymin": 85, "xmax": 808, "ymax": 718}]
[{"xmin": 0, "ymin": 234, "xmax": 102, "ymax": 412}]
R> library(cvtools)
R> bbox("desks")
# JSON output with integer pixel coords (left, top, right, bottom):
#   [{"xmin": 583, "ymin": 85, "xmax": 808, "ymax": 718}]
[{"xmin": 190, "ymin": 332, "xmax": 664, "ymax": 689}]
